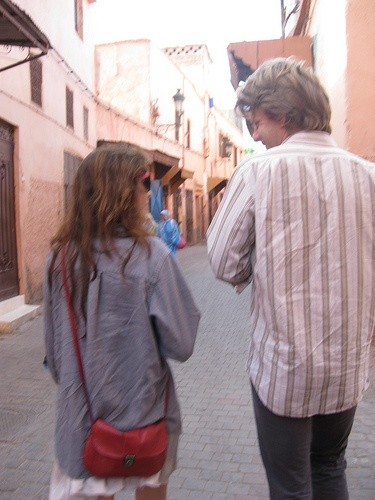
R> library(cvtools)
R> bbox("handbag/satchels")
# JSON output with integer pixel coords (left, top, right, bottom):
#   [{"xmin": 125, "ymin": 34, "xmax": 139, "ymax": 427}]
[
  {"xmin": 176, "ymin": 237, "xmax": 186, "ymax": 249},
  {"xmin": 82, "ymin": 421, "xmax": 169, "ymax": 478}
]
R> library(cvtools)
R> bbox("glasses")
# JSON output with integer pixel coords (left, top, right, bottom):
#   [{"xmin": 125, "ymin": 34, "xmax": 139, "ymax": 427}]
[{"xmin": 137, "ymin": 172, "xmax": 150, "ymax": 191}]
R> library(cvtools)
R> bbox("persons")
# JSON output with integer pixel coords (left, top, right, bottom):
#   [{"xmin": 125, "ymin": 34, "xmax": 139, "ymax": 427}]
[
  {"xmin": 156, "ymin": 209, "xmax": 180, "ymax": 255},
  {"xmin": 40, "ymin": 141, "xmax": 200, "ymax": 500},
  {"xmin": 203, "ymin": 55, "xmax": 375, "ymax": 500}
]
